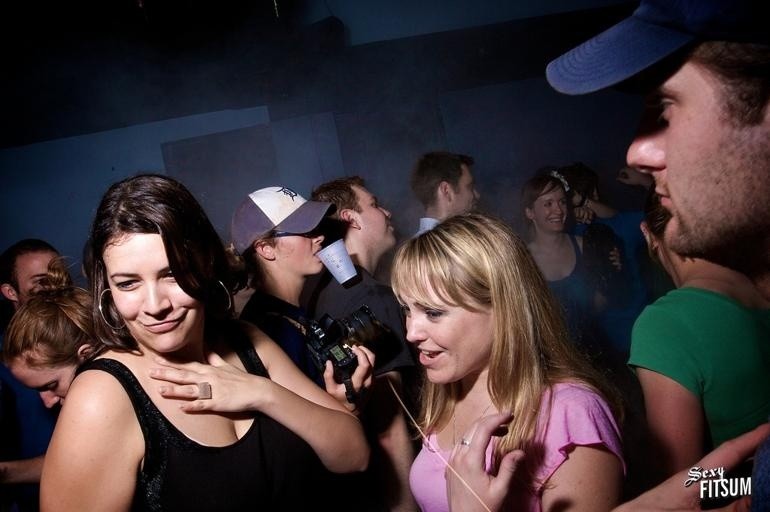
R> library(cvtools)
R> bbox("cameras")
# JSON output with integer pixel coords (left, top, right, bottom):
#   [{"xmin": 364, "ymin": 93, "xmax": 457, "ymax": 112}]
[{"xmin": 309, "ymin": 304, "xmax": 380, "ymax": 376}]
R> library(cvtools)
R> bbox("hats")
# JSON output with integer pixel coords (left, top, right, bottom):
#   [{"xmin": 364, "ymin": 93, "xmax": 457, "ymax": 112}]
[
  {"xmin": 229, "ymin": 186, "xmax": 337, "ymax": 256},
  {"xmin": 546, "ymin": 1, "xmax": 770, "ymax": 94}
]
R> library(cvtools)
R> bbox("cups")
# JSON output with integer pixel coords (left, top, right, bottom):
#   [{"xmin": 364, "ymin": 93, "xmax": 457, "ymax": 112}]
[{"xmin": 316, "ymin": 239, "xmax": 357, "ymax": 284}]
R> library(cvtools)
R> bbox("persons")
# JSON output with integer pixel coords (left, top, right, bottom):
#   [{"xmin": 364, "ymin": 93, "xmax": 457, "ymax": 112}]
[{"xmin": 0, "ymin": 0, "xmax": 770, "ymax": 512}]
